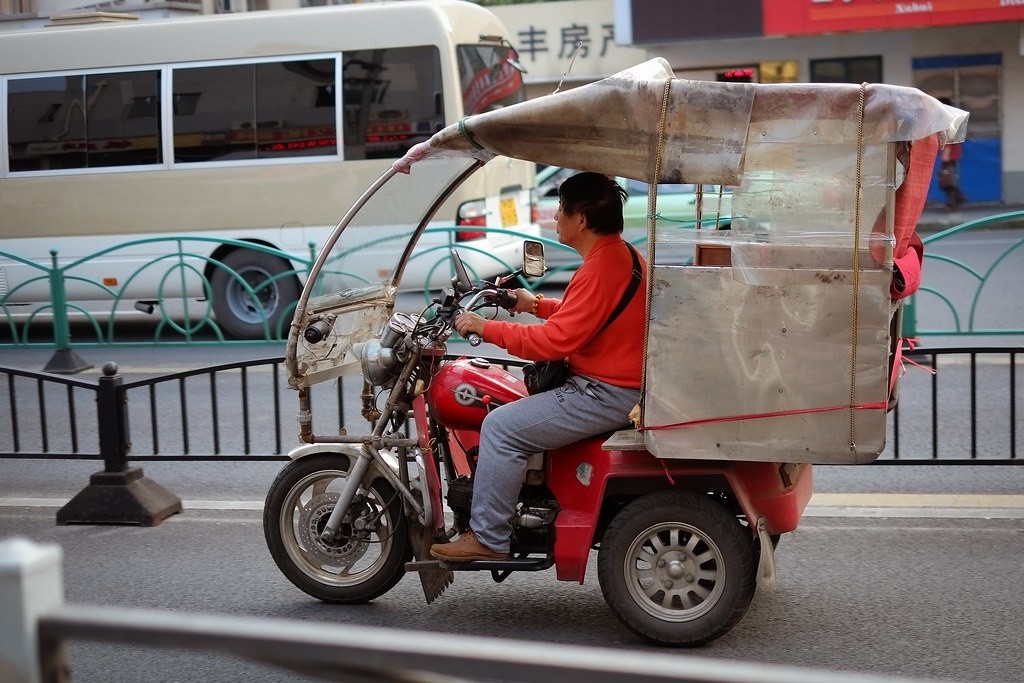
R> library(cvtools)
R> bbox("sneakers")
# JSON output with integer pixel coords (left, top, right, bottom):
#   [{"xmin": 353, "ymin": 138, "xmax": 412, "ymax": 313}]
[{"xmin": 430, "ymin": 527, "xmax": 509, "ymax": 562}]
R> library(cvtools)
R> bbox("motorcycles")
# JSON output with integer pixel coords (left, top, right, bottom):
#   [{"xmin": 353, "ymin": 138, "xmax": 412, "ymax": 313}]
[{"xmin": 260, "ymin": 71, "xmax": 948, "ymax": 649}]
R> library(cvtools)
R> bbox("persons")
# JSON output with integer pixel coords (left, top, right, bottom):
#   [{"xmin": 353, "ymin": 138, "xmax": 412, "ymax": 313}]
[
  {"xmin": 430, "ymin": 172, "xmax": 647, "ymax": 562},
  {"xmin": 937, "ymin": 96, "xmax": 962, "ymax": 212}
]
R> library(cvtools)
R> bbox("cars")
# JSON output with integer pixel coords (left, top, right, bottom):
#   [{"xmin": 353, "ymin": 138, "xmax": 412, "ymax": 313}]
[{"xmin": 535, "ymin": 163, "xmax": 850, "ymax": 271}]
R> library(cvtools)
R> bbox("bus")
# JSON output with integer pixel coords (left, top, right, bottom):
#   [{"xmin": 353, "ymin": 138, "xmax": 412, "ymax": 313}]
[{"xmin": 0, "ymin": 3, "xmax": 543, "ymax": 345}]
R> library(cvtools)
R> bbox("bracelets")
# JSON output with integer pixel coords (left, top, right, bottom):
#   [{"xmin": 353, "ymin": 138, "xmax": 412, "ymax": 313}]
[{"xmin": 532, "ymin": 293, "xmax": 544, "ymax": 318}]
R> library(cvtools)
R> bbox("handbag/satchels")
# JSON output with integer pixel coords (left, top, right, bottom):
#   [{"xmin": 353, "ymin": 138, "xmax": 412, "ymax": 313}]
[{"xmin": 522, "ymin": 359, "xmax": 569, "ymax": 395}]
